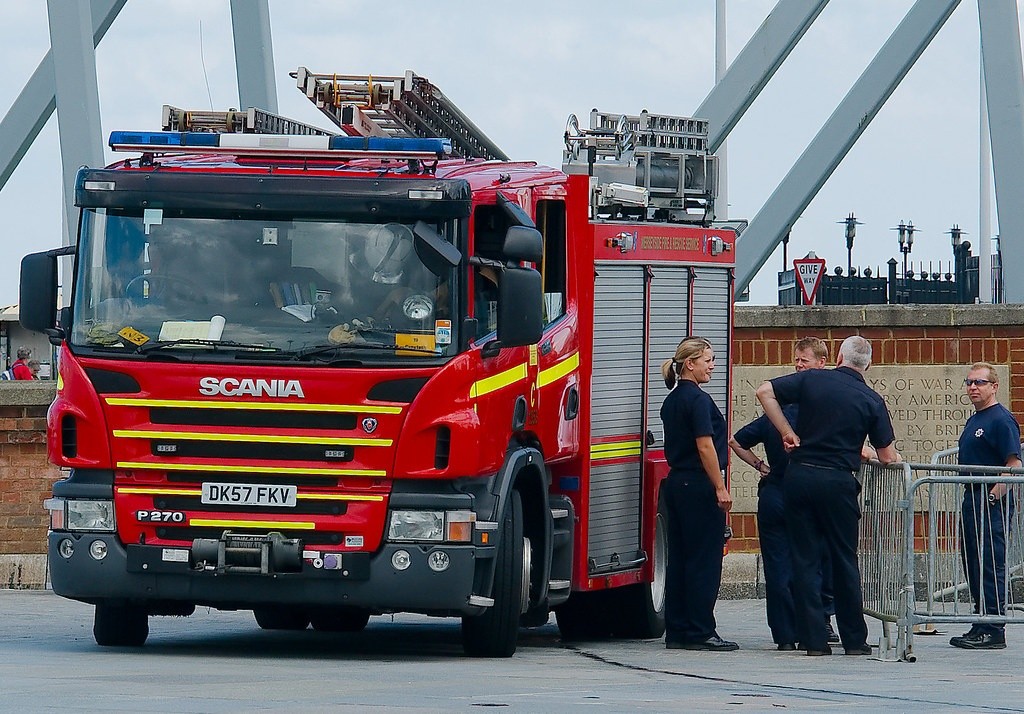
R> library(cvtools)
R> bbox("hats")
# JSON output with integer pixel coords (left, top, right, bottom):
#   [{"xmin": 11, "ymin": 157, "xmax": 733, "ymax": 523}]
[
  {"xmin": 28, "ymin": 360, "xmax": 42, "ymax": 371},
  {"xmin": 16, "ymin": 346, "xmax": 32, "ymax": 359}
]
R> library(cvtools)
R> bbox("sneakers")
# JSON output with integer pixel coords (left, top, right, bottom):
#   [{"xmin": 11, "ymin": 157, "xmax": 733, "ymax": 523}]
[
  {"xmin": 825, "ymin": 624, "xmax": 840, "ymax": 642},
  {"xmin": 950, "ymin": 625, "xmax": 1007, "ymax": 650}
]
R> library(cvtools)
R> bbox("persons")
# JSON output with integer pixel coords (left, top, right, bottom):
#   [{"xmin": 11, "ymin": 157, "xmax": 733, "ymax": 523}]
[
  {"xmin": 657, "ymin": 337, "xmax": 739, "ymax": 651},
  {"xmin": 27, "ymin": 360, "xmax": 41, "ymax": 380},
  {"xmin": 756, "ymin": 336, "xmax": 903, "ymax": 656},
  {"xmin": 374, "ymin": 250, "xmax": 499, "ymax": 328},
  {"xmin": 12, "ymin": 347, "xmax": 35, "ymax": 380},
  {"xmin": 728, "ymin": 400, "xmax": 879, "ymax": 650},
  {"xmin": 794, "ymin": 337, "xmax": 828, "ymax": 373},
  {"xmin": 950, "ymin": 362, "xmax": 1023, "ymax": 650}
]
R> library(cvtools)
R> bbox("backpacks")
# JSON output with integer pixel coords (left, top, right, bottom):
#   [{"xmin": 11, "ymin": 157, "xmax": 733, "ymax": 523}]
[{"xmin": 0, "ymin": 363, "xmax": 27, "ymax": 381}]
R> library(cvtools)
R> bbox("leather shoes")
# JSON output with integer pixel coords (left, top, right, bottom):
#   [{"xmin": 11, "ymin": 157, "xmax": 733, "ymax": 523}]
[
  {"xmin": 807, "ymin": 646, "xmax": 832, "ymax": 656},
  {"xmin": 666, "ymin": 633, "xmax": 740, "ymax": 651},
  {"xmin": 845, "ymin": 643, "xmax": 872, "ymax": 655}
]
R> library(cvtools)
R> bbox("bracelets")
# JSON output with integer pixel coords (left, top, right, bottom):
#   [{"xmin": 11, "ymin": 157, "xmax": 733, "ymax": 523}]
[{"xmin": 754, "ymin": 460, "xmax": 764, "ymax": 470}]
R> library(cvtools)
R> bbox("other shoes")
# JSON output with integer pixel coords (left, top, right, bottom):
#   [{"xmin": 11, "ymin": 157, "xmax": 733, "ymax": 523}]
[{"xmin": 777, "ymin": 642, "xmax": 808, "ymax": 650}]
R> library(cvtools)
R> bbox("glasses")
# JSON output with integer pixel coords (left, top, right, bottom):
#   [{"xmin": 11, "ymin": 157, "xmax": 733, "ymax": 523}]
[
  {"xmin": 963, "ymin": 378, "xmax": 996, "ymax": 386},
  {"xmin": 712, "ymin": 354, "xmax": 716, "ymax": 361}
]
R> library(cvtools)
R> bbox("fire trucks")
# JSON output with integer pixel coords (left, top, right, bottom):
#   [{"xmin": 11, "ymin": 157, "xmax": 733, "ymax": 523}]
[{"xmin": 19, "ymin": 65, "xmax": 738, "ymax": 657}]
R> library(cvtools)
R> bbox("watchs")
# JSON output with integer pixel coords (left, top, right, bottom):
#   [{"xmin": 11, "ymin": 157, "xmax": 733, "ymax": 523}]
[{"xmin": 988, "ymin": 493, "xmax": 998, "ymax": 503}]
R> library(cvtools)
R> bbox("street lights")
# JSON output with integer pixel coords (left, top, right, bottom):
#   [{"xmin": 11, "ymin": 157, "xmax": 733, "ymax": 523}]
[
  {"xmin": 891, "ymin": 221, "xmax": 922, "ymax": 302},
  {"xmin": 990, "ymin": 234, "xmax": 1003, "ymax": 305},
  {"xmin": 837, "ymin": 213, "xmax": 864, "ymax": 302},
  {"xmin": 782, "ymin": 216, "xmax": 800, "ymax": 273},
  {"xmin": 945, "ymin": 226, "xmax": 969, "ymax": 294}
]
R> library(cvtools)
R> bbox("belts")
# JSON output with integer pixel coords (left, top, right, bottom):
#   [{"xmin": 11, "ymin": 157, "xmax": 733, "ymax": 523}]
[{"xmin": 799, "ymin": 462, "xmax": 839, "ymax": 470}]
